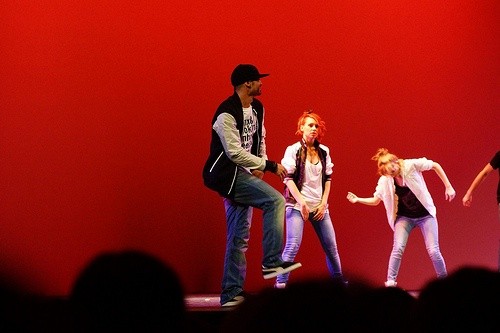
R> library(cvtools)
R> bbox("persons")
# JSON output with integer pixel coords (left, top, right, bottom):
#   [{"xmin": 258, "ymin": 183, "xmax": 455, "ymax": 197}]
[
  {"xmin": 347, "ymin": 148, "xmax": 455, "ymax": 287},
  {"xmin": 202, "ymin": 64, "xmax": 303, "ymax": 307},
  {"xmin": 1, "ymin": 245, "xmax": 479, "ymax": 333},
  {"xmin": 275, "ymin": 109, "xmax": 343, "ymax": 288},
  {"xmin": 462, "ymin": 150, "xmax": 500, "ymax": 207}
]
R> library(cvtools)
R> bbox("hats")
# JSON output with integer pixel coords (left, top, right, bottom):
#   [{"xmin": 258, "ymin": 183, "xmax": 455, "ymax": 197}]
[{"xmin": 231, "ymin": 64, "xmax": 268, "ymax": 87}]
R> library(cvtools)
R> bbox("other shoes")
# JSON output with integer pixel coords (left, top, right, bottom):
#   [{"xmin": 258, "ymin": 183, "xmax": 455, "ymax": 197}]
[
  {"xmin": 223, "ymin": 293, "xmax": 249, "ymax": 306},
  {"xmin": 262, "ymin": 261, "xmax": 301, "ymax": 279},
  {"xmin": 331, "ymin": 274, "xmax": 348, "ymax": 287},
  {"xmin": 275, "ymin": 282, "xmax": 286, "ymax": 288},
  {"xmin": 385, "ymin": 281, "xmax": 397, "ymax": 287}
]
